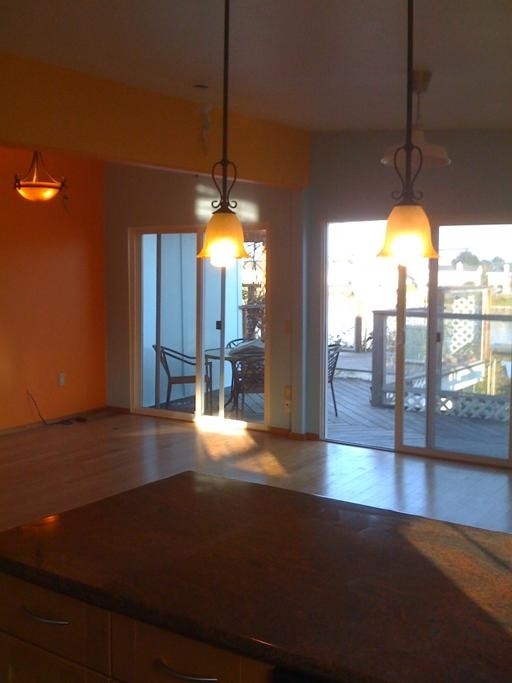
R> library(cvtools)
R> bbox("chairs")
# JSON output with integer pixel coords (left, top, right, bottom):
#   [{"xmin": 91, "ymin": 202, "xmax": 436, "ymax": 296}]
[
  {"xmin": 327, "ymin": 343, "xmax": 342, "ymax": 416},
  {"xmin": 152, "ymin": 337, "xmax": 265, "ymax": 420}
]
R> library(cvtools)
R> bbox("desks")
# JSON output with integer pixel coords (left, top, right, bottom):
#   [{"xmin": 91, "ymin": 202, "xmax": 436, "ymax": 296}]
[{"xmin": 1, "ymin": 468, "xmax": 511, "ymax": 682}]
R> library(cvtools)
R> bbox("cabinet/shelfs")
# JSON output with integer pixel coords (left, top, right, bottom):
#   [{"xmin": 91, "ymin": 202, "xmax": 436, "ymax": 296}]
[{"xmin": 1, "ymin": 572, "xmax": 274, "ymax": 683}]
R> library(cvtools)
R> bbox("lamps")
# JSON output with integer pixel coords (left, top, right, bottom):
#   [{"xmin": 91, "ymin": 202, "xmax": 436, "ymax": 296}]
[
  {"xmin": 193, "ymin": 0, "xmax": 254, "ymax": 269},
  {"xmin": 374, "ymin": 0, "xmax": 441, "ymax": 270},
  {"xmin": 13, "ymin": 150, "xmax": 66, "ymax": 204}
]
